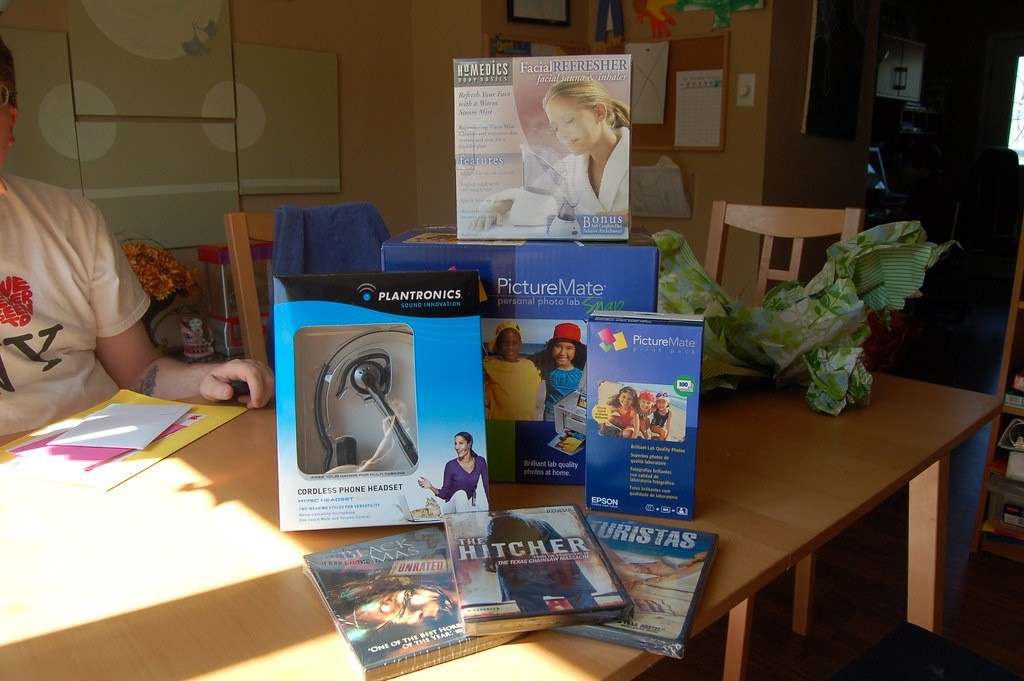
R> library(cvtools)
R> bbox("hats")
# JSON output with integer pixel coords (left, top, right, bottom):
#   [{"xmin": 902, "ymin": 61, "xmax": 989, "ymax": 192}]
[
  {"xmin": 488, "ymin": 322, "xmax": 521, "ymax": 353},
  {"xmin": 546, "ymin": 323, "xmax": 585, "ymax": 357},
  {"xmin": 656, "ymin": 395, "xmax": 669, "ymax": 402},
  {"xmin": 640, "ymin": 391, "xmax": 654, "ymax": 402}
]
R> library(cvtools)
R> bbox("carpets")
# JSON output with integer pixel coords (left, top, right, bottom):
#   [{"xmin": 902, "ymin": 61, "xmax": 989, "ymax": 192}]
[{"xmin": 825, "ymin": 621, "xmax": 1024, "ymax": 681}]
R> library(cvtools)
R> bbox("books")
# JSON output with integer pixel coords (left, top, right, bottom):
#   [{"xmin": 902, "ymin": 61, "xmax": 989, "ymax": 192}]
[{"xmin": 303, "ymin": 505, "xmax": 719, "ymax": 681}]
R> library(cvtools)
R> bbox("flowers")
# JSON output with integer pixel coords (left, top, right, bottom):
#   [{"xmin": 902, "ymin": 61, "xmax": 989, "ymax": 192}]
[{"xmin": 121, "ymin": 242, "xmax": 206, "ymax": 352}]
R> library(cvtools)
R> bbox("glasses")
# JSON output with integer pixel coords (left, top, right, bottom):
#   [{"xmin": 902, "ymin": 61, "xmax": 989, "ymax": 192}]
[
  {"xmin": 622, "ymin": 389, "xmax": 634, "ymax": 396},
  {"xmin": 0, "ymin": 85, "xmax": 18, "ymax": 107},
  {"xmin": 656, "ymin": 393, "xmax": 668, "ymax": 397}
]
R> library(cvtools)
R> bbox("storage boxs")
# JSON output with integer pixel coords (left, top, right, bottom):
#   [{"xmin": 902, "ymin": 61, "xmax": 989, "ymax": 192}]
[
  {"xmin": 585, "ymin": 308, "xmax": 703, "ymax": 519},
  {"xmin": 272, "ymin": 266, "xmax": 492, "ymax": 532},
  {"xmin": 380, "ymin": 224, "xmax": 659, "ymax": 487}
]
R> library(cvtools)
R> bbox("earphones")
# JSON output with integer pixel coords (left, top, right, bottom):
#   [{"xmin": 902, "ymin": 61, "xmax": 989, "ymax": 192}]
[{"xmin": 351, "ymin": 359, "xmax": 418, "ymax": 464}]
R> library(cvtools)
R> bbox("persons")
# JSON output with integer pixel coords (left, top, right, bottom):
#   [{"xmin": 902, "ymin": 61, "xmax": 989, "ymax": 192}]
[
  {"xmin": 418, "ymin": 431, "xmax": 489, "ymax": 506},
  {"xmin": 470, "ymin": 77, "xmax": 630, "ymax": 231},
  {"xmin": 481, "ymin": 322, "xmax": 586, "ymax": 420},
  {"xmin": 0, "ymin": 41, "xmax": 275, "ymax": 436},
  {"xmin": 597, "ymin": 387, "xmax": 671, "ymax": 441},
  {"xmin": 483, "ymin": 510, "xmax": 601, "ymax": 613}
]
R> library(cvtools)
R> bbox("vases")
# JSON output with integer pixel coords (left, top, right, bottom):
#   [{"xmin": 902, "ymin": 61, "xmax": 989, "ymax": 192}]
[{"xmin": 141, "ymin": 291, "xmax": 176, "ymax": 341}]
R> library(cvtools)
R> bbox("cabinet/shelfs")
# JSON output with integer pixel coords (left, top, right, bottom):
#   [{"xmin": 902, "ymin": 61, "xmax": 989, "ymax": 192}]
[
  {"xmin": 874, "ymin": 33, "xmax": 926, "ymax": 103},
  {"xmin": 968, "ymin": 224, "xmax": 1023, "ymax": 567}
]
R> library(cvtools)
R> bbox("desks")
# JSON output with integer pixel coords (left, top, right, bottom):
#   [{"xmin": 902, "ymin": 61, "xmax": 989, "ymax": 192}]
[{"xmin": 1, "ymin": 369, "xmax": 1001, "ymax": 681}]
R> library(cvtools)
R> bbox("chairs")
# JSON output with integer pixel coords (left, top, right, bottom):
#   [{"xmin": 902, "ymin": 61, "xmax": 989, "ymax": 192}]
[
  {"xmin": 703, "ymin": 199, "xmax": 866, "ymax": 681},
  {"xmin": 224, "ymin": 203, "xmax": 389, "ymax": 371}
]
